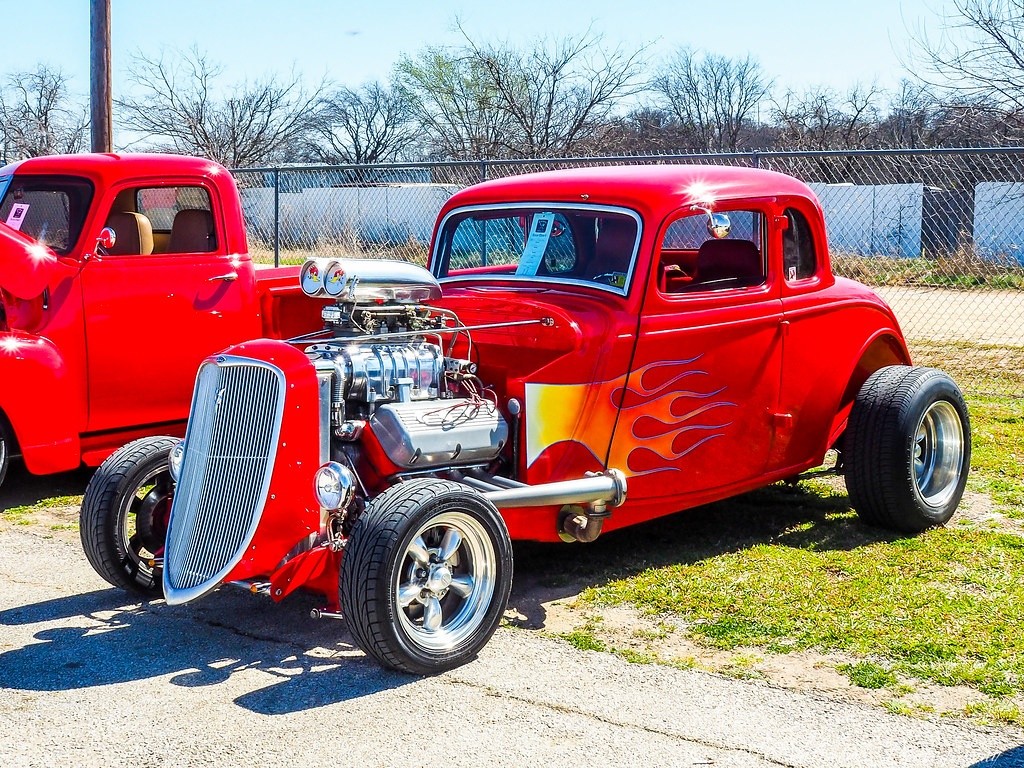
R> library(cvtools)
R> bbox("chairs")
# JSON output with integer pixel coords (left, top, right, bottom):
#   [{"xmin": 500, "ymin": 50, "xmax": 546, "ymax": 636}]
[
  {"xmin": 107, "ymin": 211, "xmax": 154, "ymax": 255},
  {"xmin": 584, "ymin": 221, "xmax": 667, "ymax": 292},
  {"xmin": 694, "ymin": 239, "xmax": 761, "ymax": 289},
  {"xmin": 166, "ymin": 209, "xmax": 216, "ymax": 254}
]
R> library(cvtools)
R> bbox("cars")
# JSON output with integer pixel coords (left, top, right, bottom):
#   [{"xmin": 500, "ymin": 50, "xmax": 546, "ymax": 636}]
[
  {"xmin": 79, "ymin": 164, "xmax": 974, "ymax": 679},
  {"xmin": 0, "ymin": 152, "xmax": 333, "ymax": 490}
]
